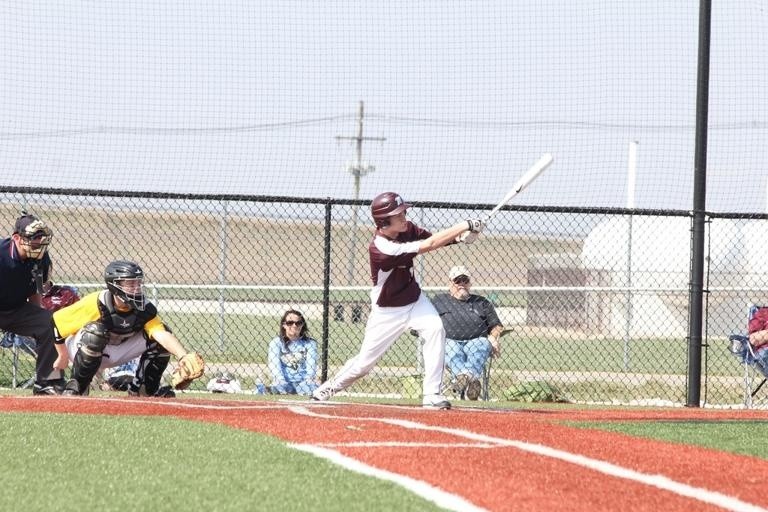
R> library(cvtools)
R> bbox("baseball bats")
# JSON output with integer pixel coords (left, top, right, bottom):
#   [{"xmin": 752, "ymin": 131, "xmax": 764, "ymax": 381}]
[{"xmin": 482, "ymin": 158, "xmax": 555, "ymax": 222}]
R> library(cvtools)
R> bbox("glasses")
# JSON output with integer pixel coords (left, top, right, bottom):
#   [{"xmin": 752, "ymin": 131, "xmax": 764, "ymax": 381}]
[
  {"xmin": 284, "ymin": 320, "xmax": 304, "ymax": 327},
  {"xmin": 453, "ymin": 277, "xmax": 470, "ymax": 284}
]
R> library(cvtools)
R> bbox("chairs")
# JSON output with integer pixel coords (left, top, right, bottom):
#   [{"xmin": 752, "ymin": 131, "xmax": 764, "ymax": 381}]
[
  {"xmin": 409, "ymin": 327, "xmax": 517, "ymax": 403},
  {"xmin": 728, "ymin": 302, "xmax": 768, "ymax": 410}
]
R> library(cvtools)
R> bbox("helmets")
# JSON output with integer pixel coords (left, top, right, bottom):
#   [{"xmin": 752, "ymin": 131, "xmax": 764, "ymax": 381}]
[
  {"xmin": 371, "ymin": 191, "xmax": 414, "ymax": 228},
  {"xmin": 103, "ymin": 260, "xmax": 147, "ymax": 312}
]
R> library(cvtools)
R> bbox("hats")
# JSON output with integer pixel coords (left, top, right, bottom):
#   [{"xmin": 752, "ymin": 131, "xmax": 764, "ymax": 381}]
[
  {"xmin": 12, "ymin": 215, "xmax": 53, "ymax": 261},
  {"xmin": 448, "ymin": 265, "xmax": 472, "ymax": 282}
]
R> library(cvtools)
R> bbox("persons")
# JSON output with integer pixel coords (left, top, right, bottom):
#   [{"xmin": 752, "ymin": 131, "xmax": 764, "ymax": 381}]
[
  {"xmin": 253, "ymin": 309, "xmax": 320, "ymax": 397},
  {"xmin": 431, "ymin": 265, "xmax": 504, "ymax": 401},
  {"xmin": 306, "ymin": 191, "xmax": 486, "ymax": 411},
  {"xmin": 748, "ymin": 305, "xmax": 768, "ymax": 379},
  {"xmin": 0, "ymin": 214, "xmax": 66, "ymax": 395},
  {"xmin": 49, "ymin": 260, "xmax": 205, "ymax": 398}
]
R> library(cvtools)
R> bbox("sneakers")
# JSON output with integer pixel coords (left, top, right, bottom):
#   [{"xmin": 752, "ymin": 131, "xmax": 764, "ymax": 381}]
[
  {"xmin": 32, "ymin": 376, "xmax": 67, "ymax": 396},
  {"xmin": 312, "ymin": 383, "xmax": 336, "ymax": 401},
  {"xmin": 450, "ymin": 373, "xmax": 482, "ymax": 401},
  {"xmin": 61, "ymin": 378, "xmax": 89, "ymax": 396},
  {"xmin": 422, "ymin": 394, "xmax": 452, "ymax": 410},
  {"xmin": 128, "ymin": 386, "xmax": 176, "ymax": 398}
]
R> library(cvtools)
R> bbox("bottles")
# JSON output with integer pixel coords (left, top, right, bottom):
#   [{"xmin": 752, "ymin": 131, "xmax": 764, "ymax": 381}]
[
  {"xmin": 216, "ymin": 376, "xmax": 229, "ymax": 385},
  {"xmin": 255, "ymin": 374, "xmax": 266, "ymax": 395}
]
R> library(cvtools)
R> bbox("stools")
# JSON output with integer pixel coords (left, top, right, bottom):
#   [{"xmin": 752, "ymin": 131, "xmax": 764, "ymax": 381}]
[{"xmin": 0, "ymin": 327, "xmax": 41, "ymax": 391}]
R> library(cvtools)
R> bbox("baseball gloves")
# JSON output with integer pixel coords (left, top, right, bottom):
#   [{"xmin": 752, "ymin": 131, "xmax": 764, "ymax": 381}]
[{"xmin": 172, "ymin": 351, "xmax": 205, "ymax": 390}]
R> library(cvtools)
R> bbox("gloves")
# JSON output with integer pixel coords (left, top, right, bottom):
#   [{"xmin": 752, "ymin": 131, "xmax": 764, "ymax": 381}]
[
  {"xmin": 466, "ymin": 217, "xmax": 484, "ymax": 233},
  {"xmin": 459, "ymin": 231, "xmax": 478, "ymax": 244}
]
